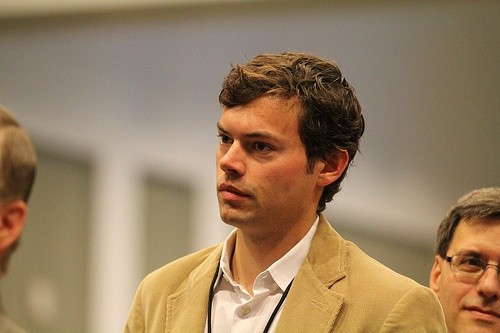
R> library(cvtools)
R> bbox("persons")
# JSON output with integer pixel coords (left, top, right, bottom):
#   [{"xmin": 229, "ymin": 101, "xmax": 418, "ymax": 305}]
[
  {"xmin": 0, "ymin": 101, "xmax": 41, "ymax": 332},
  {"xmin": 124, "ymin": 52, "xmax": 450, "ymax": 333},
  {"xmin": 428, "ymin": 186, "xmax": 499, "ymax": 333}
]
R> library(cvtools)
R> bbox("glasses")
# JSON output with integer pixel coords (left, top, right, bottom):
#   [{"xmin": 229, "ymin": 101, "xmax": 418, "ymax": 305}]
[{"xmin": 441, "ymin": 254, "xmax": 500, "ymax": 275}]
